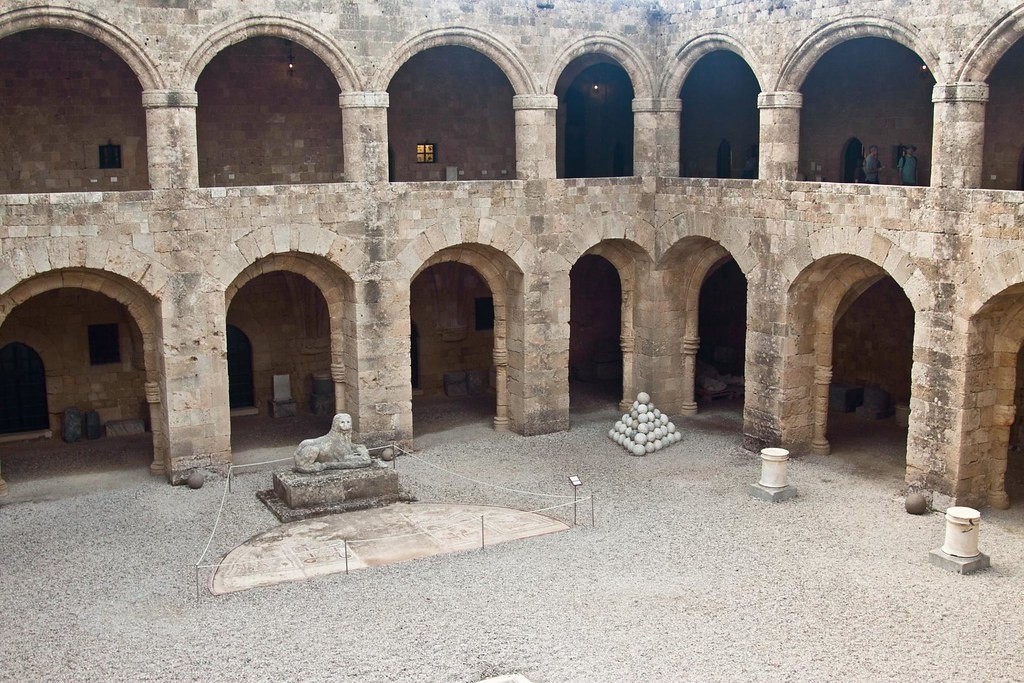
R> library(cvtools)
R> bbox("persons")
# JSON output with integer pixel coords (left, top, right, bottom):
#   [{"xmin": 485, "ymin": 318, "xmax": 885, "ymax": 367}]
[
  {"xmin": 896, "ymin": 145, "xmax": 917, "ymax": 185},
  {"xmin": 854, "ymin": 144, "xmax": 885, "ymax": 184}
]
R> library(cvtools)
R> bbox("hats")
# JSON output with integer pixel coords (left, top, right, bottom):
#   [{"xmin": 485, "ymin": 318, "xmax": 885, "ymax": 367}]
[{"xmin": 905, "ymin": 144, "xmax": 917, "ymax": 152}]
